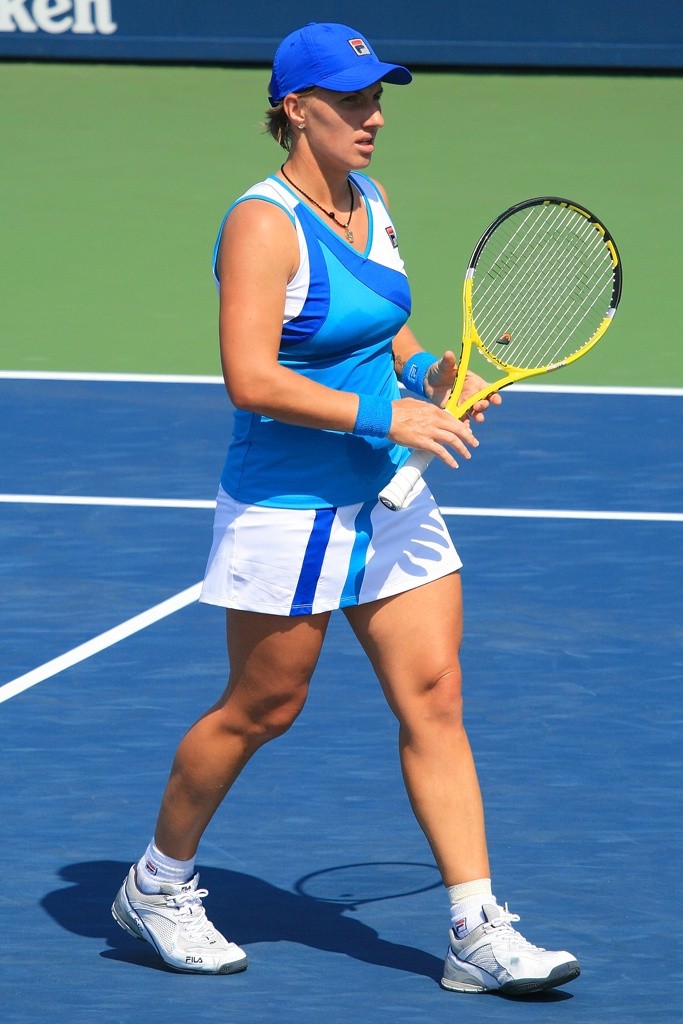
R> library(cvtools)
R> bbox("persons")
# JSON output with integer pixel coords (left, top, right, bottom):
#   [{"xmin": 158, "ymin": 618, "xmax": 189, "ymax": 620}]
[{"xmin": 112, "ymin": 23, "xmax": 582, "ymax": 996}]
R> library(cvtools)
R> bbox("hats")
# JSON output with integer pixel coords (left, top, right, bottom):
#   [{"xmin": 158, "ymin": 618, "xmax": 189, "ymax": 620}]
[{"xmin": 268, "ymin": 22, "xmax": 413, "ymax": 108}]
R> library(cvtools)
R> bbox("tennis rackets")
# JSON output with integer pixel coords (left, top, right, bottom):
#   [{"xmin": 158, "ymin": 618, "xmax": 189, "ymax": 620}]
[{"xmin": 378, "ymin": 197, "xmax": 623, "ymax": 510}]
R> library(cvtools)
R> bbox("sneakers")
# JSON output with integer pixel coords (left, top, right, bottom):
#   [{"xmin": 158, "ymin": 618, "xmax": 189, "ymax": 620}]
[
  {"xmin": 441, "ymin": 903, "xmax": 580, "ymax": 994},
  {"xmin": 111, "ymin": 863, "xmax": 249, "ymax": 974}
]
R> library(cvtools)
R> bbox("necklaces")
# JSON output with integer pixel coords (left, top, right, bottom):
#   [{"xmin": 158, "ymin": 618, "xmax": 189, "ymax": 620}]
[{"xmin": 281, "ymin": 164, "xmax": 355, "ymax": 243}]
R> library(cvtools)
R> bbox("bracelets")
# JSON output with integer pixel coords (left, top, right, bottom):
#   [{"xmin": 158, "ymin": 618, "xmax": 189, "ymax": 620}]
[
  {"xmin": 402, "ymin": 351, "xmax": 439, "ymax": 399},
  {"xmin": 354, "ymin": 392, "xmax": 393, "ymax": 440}
]
